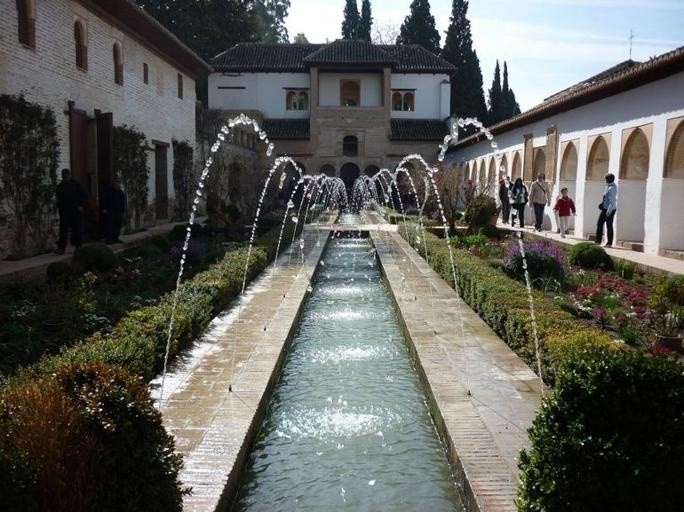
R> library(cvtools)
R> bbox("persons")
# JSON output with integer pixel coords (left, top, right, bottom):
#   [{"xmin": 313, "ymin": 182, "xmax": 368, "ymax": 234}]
[
  {"xmin": 498, "ymin": 179, "xmax": 510, "ymax": 225},
  {"xmin": 101, "ymin": 178, "xmax": 124, "ymax": 244},
  {"xmin": 593, "ymin": 173, "xmax": 617, "ymax": 245},
  {"xmin": 509, "ymin": 177, "xmax": 528, "ymax": 228},
  {"xmin": 528, "ymin": 173, "xmax": 550, "ymax": 231},
  {"xmin": 505, "ymin": 176, "xmax": 511, "ymax": 191},
  {"xmin": 553, "ymin": 188, "xmax": 576, "ymax": 238},
  {"xmin": 53, "ymin": 168, "xmax": 87, "ymax": 253}
]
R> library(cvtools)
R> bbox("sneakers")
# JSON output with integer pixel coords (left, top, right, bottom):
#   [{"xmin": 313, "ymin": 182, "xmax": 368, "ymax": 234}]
[{"xmin": 501, "ymin": 219, "xmax": 612, "ymax": 249}]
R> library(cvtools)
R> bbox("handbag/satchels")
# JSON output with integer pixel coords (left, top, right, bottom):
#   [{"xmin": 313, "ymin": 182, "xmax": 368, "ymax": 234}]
[{"xmin": 598, "ymin": 203, "xmax": 603, "ymax": 210}]
